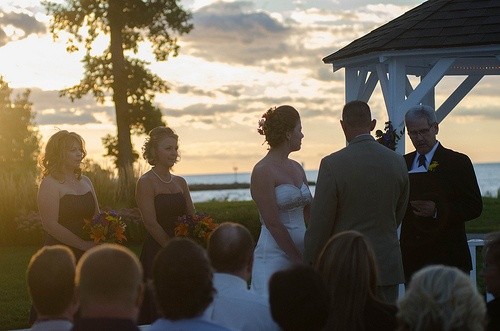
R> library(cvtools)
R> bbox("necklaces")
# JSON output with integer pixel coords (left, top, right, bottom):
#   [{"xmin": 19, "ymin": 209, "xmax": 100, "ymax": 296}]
[{"xmin": 152, "ymin": 168, "xmax": 175, "ymax": 183}]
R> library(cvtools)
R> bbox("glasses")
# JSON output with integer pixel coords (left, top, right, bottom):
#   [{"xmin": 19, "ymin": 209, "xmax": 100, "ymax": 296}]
[{"xmin": 407, "ymin": 121, "xmax": 436, "ymax": 138}]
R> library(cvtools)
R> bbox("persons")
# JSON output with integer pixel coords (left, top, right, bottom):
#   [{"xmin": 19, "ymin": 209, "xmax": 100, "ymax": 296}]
[
  {"xmin": 304, "ymin": 98, "xmax": 410, "ymax": 305},
  {"xmin": 20, "ymin": 241, "xmax": 500, "ymax": 331},
  {"xmin": 138, "ymin": 123, "xmax": 198, "ymax": 269},
  {"xmin": 398, "ymin": 106, "xmax": 483, "ymax": 296},
  {"xmin": 249, "ymin": 100, "xmax": 314, "ymax": 290},
  {"xmin": 35, "ymin": 130, "xmax": 105, "ymax": 266}
]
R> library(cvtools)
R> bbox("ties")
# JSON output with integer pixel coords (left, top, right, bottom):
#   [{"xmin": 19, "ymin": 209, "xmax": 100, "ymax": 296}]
[{"xmin": 415, "ymin": 154, "xmax": 426, "ymax": 171}]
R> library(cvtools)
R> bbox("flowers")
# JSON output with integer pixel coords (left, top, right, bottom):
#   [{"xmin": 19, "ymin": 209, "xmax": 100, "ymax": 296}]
[
  {"xmin": 424, "ymin": 160, "xmax": 441, "ymax": 180},
  {"xmin": 81, "ymin": 206, "xmax": 128, "ymax": 246},
  {"xmin": 375, "ymin": 119, "xmax": 405, "ymax": 151},
  {"xmin": 173, "ymin": 208, "xmax": 220, "ymax": 246},
  {"xmin": 257, "ymin": 106, "xmax": 277, "ymax": 146}
]
